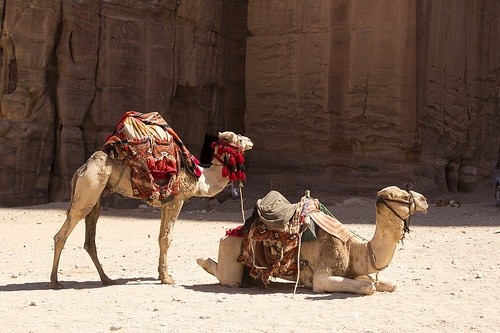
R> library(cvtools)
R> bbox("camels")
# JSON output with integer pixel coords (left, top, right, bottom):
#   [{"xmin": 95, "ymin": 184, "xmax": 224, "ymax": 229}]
[
  {"xmin": 50, "ymin": 112, "xmax": 253, "ymax": 285},
  {"xmin": 198, "ymin": 186, "xmax": 428, "ymax": 293}
]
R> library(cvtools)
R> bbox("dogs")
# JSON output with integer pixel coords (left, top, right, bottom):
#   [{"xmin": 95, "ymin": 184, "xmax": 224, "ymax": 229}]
[{"xmin": 431, "ymin": 198, "xmax": 461, "ymax": 208}]
